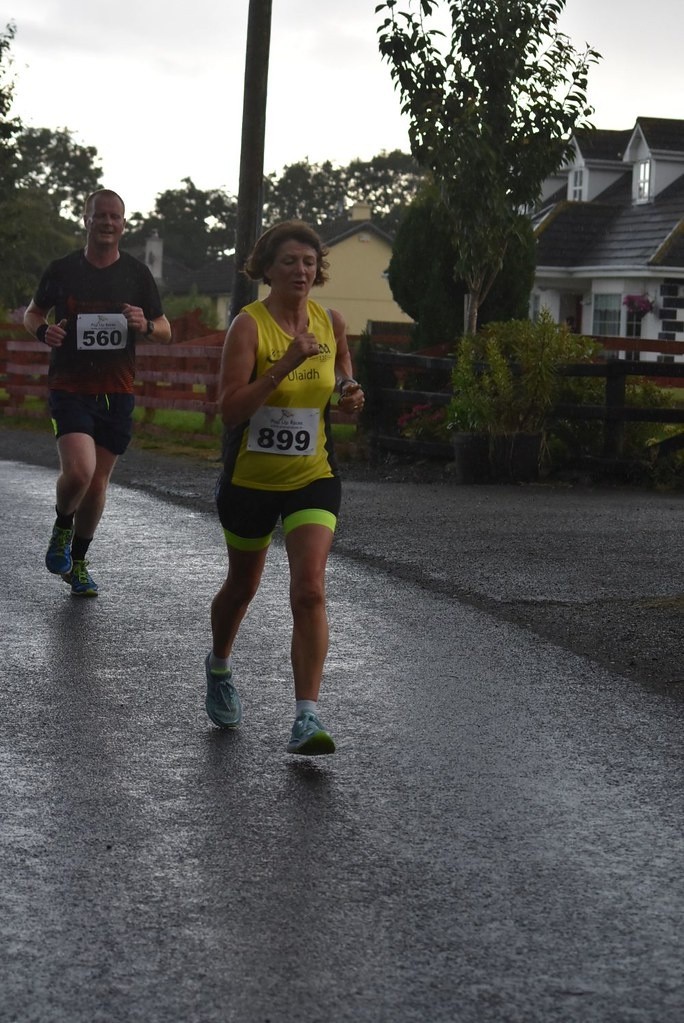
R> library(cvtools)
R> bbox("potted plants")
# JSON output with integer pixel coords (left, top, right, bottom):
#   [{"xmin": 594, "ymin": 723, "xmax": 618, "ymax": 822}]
[{"xmin": 446, "ymin": 302, "xmax": 605, "ymax": 487}]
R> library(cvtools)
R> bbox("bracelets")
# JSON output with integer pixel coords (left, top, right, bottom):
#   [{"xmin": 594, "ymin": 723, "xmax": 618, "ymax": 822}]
[
  {"xmin": 263, "ymin": 369, "xmax": 278, "ymax": 388},
  {"xmin": 338, "ymin": 378, "xmax": 357, "ymax": 395}
]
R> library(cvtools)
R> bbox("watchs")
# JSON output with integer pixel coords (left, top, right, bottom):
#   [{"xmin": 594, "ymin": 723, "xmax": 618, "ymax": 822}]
[{"xmin": 144, "ymin": 320, "xmax": 155, "ymax": 336}]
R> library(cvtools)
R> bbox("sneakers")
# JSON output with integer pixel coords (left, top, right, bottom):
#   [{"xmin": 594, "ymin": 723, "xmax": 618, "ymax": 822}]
[
  {"xmin": 285, "ymin": 711, "xmax": 335, "ymax": 756},
  {"xmin": 204, "ymin": 650, "xmax": 241, "ymax": 727},
  {"xmin": 62, "ymin": 554, "xmax": 98, "ymax": 596},
  {"xmin": 45, "ymin": 523, "xmax": 74, "ymax": 573}
]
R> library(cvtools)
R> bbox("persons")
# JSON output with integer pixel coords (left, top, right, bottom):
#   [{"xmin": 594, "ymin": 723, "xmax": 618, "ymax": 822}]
[
  {"xmin": 203, "ymin": 221, "xmax": 366, "ymax": 756},
  {"xmin": 22, "ymin": 188, "xmax": 172, "ymax": 596}
]
